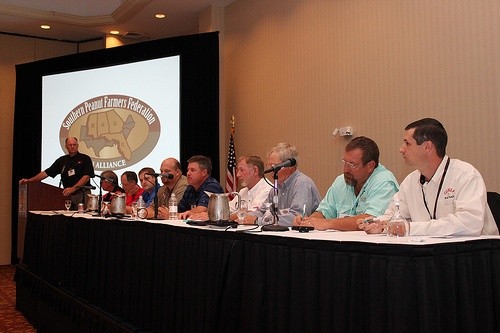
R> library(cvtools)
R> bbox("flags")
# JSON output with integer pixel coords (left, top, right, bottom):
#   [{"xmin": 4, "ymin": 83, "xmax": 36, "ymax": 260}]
[{"xmin": 226, "ymin": 127, "xmax": 238, "ymax": 201}]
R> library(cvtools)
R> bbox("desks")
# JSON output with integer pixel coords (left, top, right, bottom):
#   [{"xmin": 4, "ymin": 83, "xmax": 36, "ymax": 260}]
[{"xmin": 15, "ymin": 211, "xmax": 500, "ymax": 333}]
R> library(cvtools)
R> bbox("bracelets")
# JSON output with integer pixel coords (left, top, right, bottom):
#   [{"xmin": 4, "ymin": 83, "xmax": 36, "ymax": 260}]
[{"xmin": 254, "ymin": 217, "xmax": 258, "ymax": 224}]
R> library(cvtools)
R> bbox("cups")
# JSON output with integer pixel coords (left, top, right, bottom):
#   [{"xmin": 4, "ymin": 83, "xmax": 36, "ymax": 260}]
[{"xmin": 78, "ymin": 203, "xmax": 84, "ymax": 215}]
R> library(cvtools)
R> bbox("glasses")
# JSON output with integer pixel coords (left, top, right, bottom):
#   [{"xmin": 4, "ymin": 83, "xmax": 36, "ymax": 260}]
[
  {"xmin": 342, "ymin": 158, "xmax": 369, "ymax": 169},
  {"xmin": 266, "ymin": 164, "xmax": 275, "ymax": 170},
  {"xmin": 120, "ymin": 180, "xmax": 134, "ymax": 187}
]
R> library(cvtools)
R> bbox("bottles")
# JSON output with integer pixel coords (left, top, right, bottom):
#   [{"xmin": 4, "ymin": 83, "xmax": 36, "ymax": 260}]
[
  {"xmin": 169, "ymin": 193, "xmax": 178, "ymax": 222},
  {"xmin": 136, "ymin": 196, "xmax": 146, "ymax": 220}
]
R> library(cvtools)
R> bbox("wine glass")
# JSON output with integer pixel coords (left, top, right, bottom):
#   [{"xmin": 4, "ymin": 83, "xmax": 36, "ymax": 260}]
[
  {"xmin": 261, "ymin": 202, "xmax": 275, "ymax": 226},
  {"xmin": 132, "ymin": 202, "xmax": 141, "ymax": 221},
  {"xmin": 65, "ymin": 200, "xmax": 71, "ymax": 213},
  {"xmin": 386, "ymin": 198, "xmax": 410, "ymax": 240}
]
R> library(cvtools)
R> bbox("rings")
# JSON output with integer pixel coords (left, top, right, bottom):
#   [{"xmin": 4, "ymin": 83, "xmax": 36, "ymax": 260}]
[{"xmin": 396, "ymin": 225, "xmax": 398, "ymax": 229}]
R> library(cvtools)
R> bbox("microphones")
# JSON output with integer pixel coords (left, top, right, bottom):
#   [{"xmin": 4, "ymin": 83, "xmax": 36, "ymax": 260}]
[
  {"xmin": 96, "ymin": 175, "xmax": 116, "ymax": 184},
  {"xmin": 264, "ymin": 158, "xmax": 296, "ymax": 174},
  {"xmin": 145, "ymin": 173, "xmax": 174, "ymax": 179},
  {"xmin": 61, "ymin": 163, "xmax": 66, "ymax": 173},
  {"xmin": 76, "ymin": 186, "xmax": 96, "ymax": 190}
]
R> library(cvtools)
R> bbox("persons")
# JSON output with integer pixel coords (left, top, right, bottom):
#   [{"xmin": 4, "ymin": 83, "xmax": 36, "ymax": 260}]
[
  {"xmin": 294, "ymin": 136, "xmax": 400, "ymax": 231},
  {"xmin": 143, "ymin": 158, "xmax": 188, "ymax": 218},
  {"xmin": 158, "ymin": 156, "xmax": 224, "ymax": 220},
  {"xmin": 356, "ymin": 118, "xmax": 499, "ymax": 238},
  {"xmin": 20, "ymin": 137, "xmax": 95, "ymax": 211},
  {"xmin": 100, "ymin": 170, "xmax": 125, "ymax": 204},
  {"xmin": 191, "ymin": 155, "xmax": 274, "ymax": 221},
  {"xmin": 126, "ymin": 168, "xmax": 161, "ymax": 213},
  {"xmin": 107, "ymin": 171, "xmax": 143, "ymax": 213},
  {"xmin": 230, "ymin": 143, "xmax": 321, "ymax": 226}
]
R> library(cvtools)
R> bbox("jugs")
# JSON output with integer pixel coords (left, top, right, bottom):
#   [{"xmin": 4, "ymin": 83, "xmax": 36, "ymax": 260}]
[
  {"xmin": 86, "ymin": 193, "xmax": 99, "ymax": 216},
  {"xmin": 110, "ymin": 192, "xmax": 126, "ymax": 214},
  {"xmin": 204, "ymin": 190, "xmax": 241, "ymax": 223}
]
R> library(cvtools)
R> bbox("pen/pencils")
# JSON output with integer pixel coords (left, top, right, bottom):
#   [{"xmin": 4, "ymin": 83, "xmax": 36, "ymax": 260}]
[
  {"xmin": 364, "ymin": 219, "xmax": 373, "ymax": 223},
  {"xmin": 302, "ymin": 204, "xmax": 306, "ymax": 218}
]
